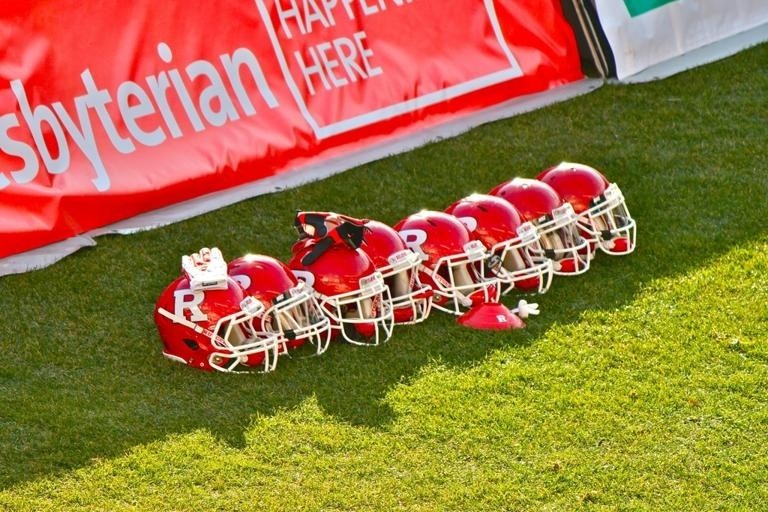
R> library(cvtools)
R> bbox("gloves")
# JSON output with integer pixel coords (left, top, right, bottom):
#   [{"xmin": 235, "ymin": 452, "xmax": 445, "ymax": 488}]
[
  {"xmin": 293, "ymin": 211, "xmax": 363, "ymax": 265},
  {"xmin": 181, "ymin": 247, "xmax": 228, "ymax": 291}
]
